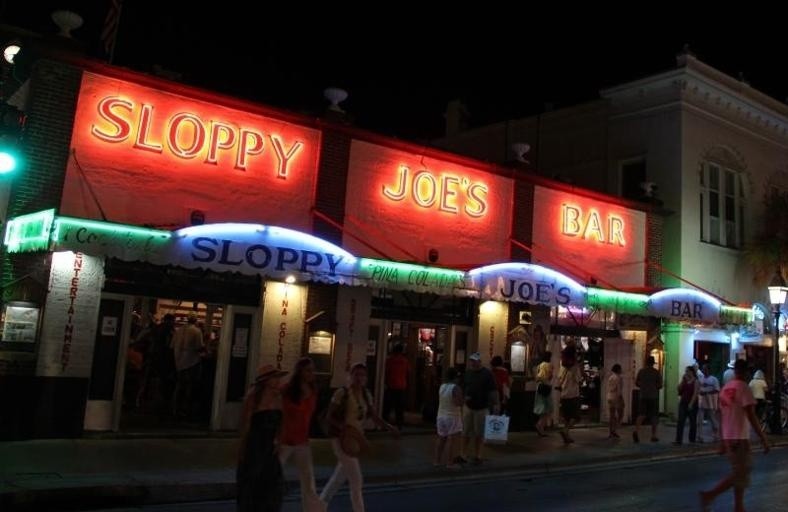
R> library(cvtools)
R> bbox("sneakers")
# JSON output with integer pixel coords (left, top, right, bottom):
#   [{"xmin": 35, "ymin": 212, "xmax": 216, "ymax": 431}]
[{"xmin": 535, "ymin": 424, "xmax": 698, "ymax": 449}]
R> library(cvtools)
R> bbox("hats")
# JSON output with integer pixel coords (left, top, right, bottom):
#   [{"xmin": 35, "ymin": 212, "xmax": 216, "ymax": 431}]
[
  {"xmin": 351, "ymin": 361, "xmax": 367, "ymax": 373},
  {"xmin": 337, "ymin": 423, "xmax": 370, "ymax": 458},
  {"xmin": 468, "ymin": 351, "xmax": 481, "ymax": 361},
  {"xmin": 249, "ymin": 363, "xmax": 290, "ymax": 388}
]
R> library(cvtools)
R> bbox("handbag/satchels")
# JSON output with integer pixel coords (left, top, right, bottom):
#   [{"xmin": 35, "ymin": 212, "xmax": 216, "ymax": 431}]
[
  {"xmin": 483, "ymin": 409, "xmax": 510, "ymax": 444},
  {"xmin": 319, "ymin": 385, "xmax": 349, "ymax": 437},
  {"xmin": 537, "ymin": 383, "xmax": 552, "ymax": 398}
]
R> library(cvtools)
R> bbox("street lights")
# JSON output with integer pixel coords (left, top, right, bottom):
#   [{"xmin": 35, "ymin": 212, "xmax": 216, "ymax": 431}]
[{"xmin": 767, "ymin": 264, "xmax": 788, "ymax": 436}]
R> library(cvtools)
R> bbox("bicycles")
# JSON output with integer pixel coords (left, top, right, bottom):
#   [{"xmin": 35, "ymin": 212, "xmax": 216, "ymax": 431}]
[{"xmin": 758, "ymin": 399, "xmax": 788, "ymax": 437}]
